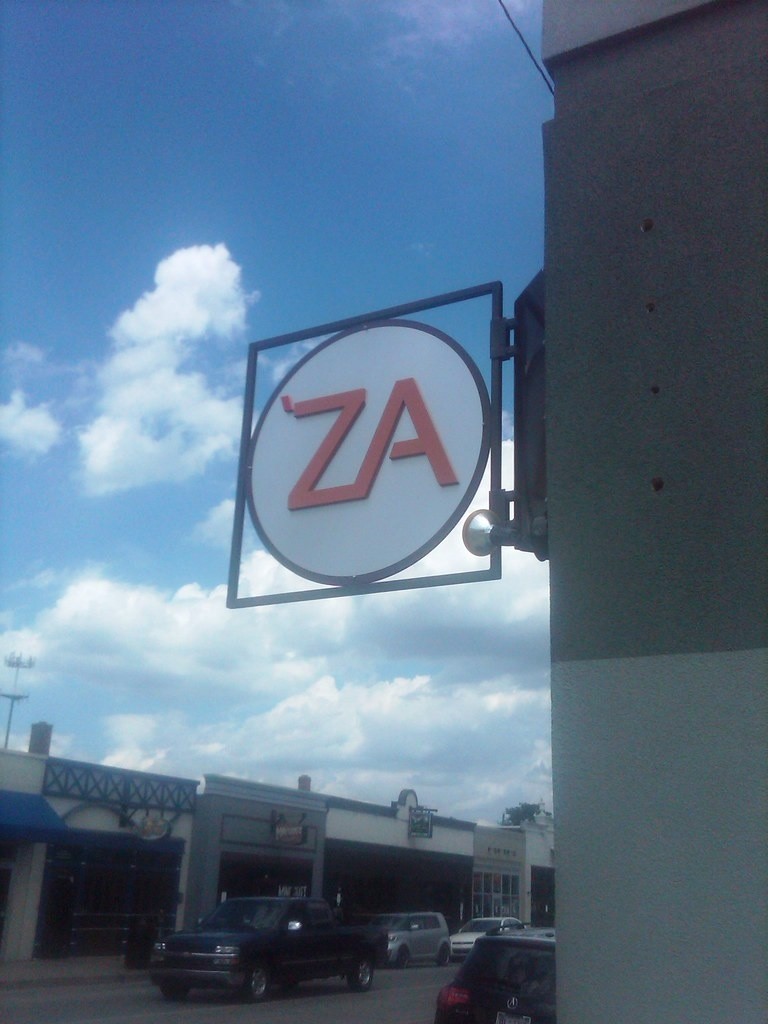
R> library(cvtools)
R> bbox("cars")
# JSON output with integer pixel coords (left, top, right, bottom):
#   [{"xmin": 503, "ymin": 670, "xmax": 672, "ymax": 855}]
[
  {"xmin": 450, "ymin": 917, "xmax": 527, "ymax": 956},
  {"xmin": 371, "ymin": 912, "xmax": 452, "ymax": 968}
]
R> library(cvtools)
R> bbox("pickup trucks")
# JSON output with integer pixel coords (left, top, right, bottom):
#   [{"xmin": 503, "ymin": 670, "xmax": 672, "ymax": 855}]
[{"xmin": 144, "ymin": 896, "xmax": 389, "ymax": 1001}]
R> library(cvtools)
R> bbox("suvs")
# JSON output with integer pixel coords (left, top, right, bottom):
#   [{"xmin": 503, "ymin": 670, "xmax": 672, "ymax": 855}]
[{"xmin": 432, "ymin": 921, "xmax": 557, "ymax": 1024}]
